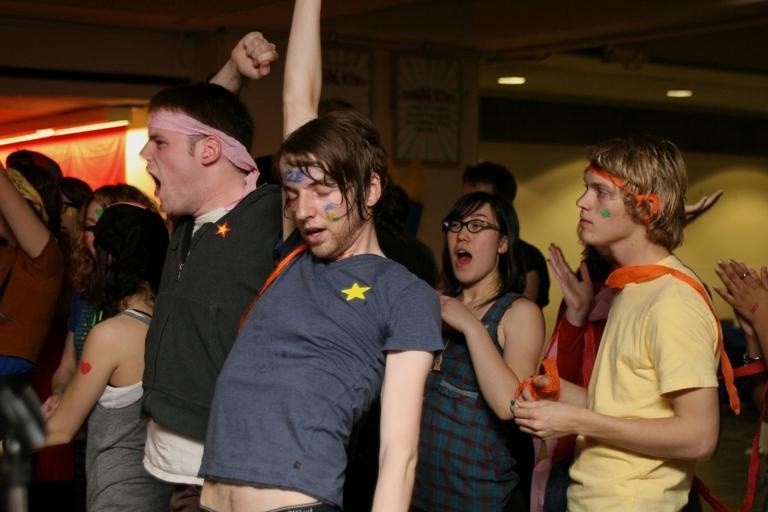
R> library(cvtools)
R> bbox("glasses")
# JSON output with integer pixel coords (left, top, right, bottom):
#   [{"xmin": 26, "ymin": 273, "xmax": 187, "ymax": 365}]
[
  {"xmin": 442, "ymin": 218, "xmax": 499, "ymax": 234},
  {"xmin": 59, "ymin": 201, "xmax": 78, "ymax": 213}
]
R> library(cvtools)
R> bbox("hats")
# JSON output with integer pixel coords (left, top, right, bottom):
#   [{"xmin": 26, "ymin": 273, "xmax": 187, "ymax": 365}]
[
  {"xmin": 83, "ymin": 205, "xmax": 169, "ymax": 255},
  {"xmin": 6, "ymin": 149, "xmax": 63, "ymax": 185}
]
